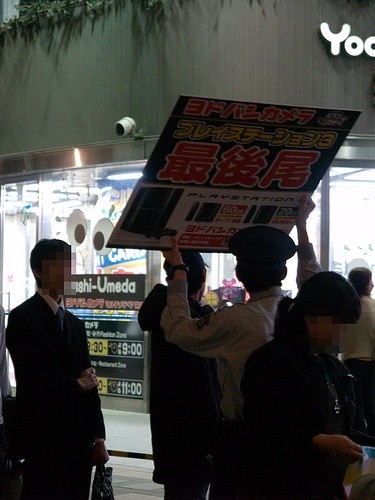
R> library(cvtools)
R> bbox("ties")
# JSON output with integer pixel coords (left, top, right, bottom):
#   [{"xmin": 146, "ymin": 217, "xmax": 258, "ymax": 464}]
[{"xmin": 56, "ymin": 306, "xmax": 63, "ymax": 333}]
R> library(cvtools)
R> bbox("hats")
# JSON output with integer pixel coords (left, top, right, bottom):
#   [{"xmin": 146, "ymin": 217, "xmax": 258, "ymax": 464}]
[
  {"xmin": 227, "ymin": 225, "xmax": 296, "ymax": 270},
  {"xmin": 163, "ymin": 248, "xmax": 210, "ymax": 278}
]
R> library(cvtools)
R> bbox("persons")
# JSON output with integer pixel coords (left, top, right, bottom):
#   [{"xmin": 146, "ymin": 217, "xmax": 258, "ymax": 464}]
[
  {"xmin": 6, "ymin": 238, "xmax": 110, "ymax": 500},
  {"xmin": 161, "ymin": 193, "xmax": 324, "ymax": 500},
  {"xmin": 336, "ymin": 267, "xmax": 375, "ymax": 447},
  {"xmin": 237, "ymin": 271, "xmax": 375, "ymax": 500},
  {"xmin": 0, "ymin": 304, "xmax": 11, "ymax": 500},
  {"xmin": 137, "ymin": 248, "xmax": 224, "ymax": 500}
]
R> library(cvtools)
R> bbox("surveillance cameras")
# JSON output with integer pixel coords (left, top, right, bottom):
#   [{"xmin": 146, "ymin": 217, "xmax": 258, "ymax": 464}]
[{"xmin": 114, "ymin": 117, "xmax": 136, "ymax": 137}]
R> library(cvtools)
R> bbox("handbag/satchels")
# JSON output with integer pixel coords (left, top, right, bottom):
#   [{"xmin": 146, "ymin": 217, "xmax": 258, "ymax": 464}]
[{"xmin": 91, "ymin": 459, "xmax": 115, "ymax": 499}]
